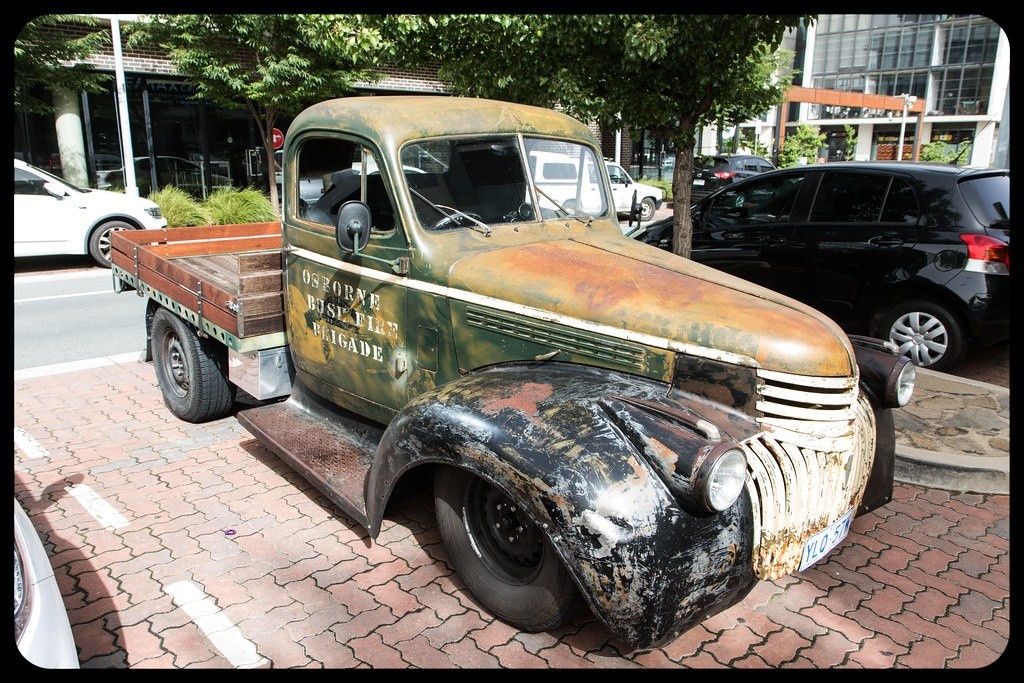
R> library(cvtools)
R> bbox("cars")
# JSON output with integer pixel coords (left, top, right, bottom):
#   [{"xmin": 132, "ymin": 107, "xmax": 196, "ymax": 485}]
[
  {"xmin": 271, "ymin": 149, "xmax": 429, "ymax": 219},
  {"xmin": 524, "ymin": 149, "xmax": 589, "ymax": 217},
  {"xmin": 14, "ymin": 157, "xmax": 169, "ymax": 271}
]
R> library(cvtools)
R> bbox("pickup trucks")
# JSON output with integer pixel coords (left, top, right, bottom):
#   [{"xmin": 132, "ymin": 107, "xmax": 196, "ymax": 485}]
[{"xmin": 101, "ymin": 91, "xmax": 917, "ymax": 653}]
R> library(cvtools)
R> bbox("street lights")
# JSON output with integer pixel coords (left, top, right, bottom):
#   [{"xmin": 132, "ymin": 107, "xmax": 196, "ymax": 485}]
[{"xmin": 891, "ymin": 92, "xmax": 918, "ymax": 162}]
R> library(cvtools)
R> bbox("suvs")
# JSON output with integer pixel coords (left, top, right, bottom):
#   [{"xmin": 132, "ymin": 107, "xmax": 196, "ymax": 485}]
[
  {"xmin": 688, "ymin": 152, "xmax": 794, "ymax": 210},
  {"xmin": 624, "ymin": 158, "xmax": 1010, "ymax": 372},
  {"xmin": 575, "ymin": 157, "xmax": 666, "ymax": 223}
]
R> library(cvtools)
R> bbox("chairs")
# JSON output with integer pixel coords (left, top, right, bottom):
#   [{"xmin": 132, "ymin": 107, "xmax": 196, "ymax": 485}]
[
  {"xmin": 394, "ymin": 172, "xmax": 464, "ymax": 234},
  {"xmin": 471, "ymin": 163, "xmax": 528, "ymax": 225}
]
